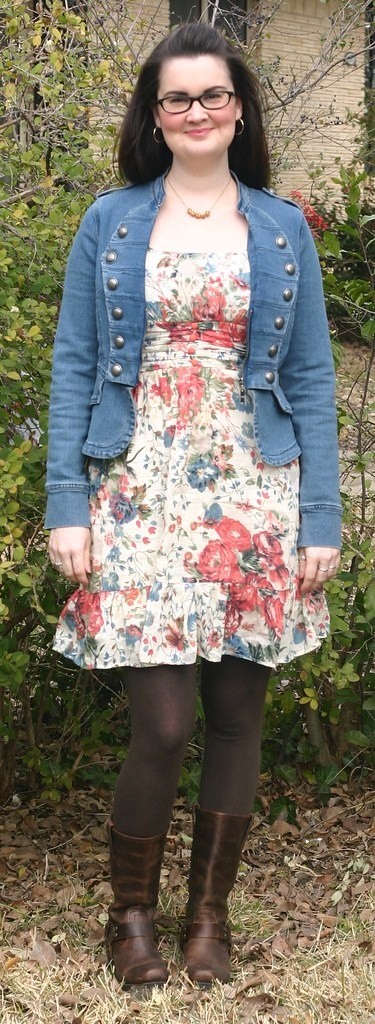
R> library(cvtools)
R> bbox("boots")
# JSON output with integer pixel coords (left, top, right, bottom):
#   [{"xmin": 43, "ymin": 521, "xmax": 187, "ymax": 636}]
[
  {"xmin": 186, "ymin": 803, "xmax": 254, "ymax": 989},
  {"xmin": 105, "ymin": 813, "xmax": 170, "ymax": 987}
]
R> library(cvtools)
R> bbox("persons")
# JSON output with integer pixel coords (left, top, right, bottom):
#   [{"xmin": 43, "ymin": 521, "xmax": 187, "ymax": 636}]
[{"xmin": 44, "ymin": 22, "xmax": 341, "ymax": 991}]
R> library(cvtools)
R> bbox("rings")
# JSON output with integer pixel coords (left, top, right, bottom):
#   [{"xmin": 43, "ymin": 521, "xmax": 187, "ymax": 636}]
[
  {"xmin": 317, "ymin": 568, "xmax": 328, "ymax": 572},
  {"xmin": 328, "ymin": 565, "xmax": 335, "ymax": 569},
  {"xmin": 298, "ymin": 556, "xmax": 306, "ymax": 561},
  {"xmin": 54, "ymin": 562, "xmax": 62, "ymax": 568}
]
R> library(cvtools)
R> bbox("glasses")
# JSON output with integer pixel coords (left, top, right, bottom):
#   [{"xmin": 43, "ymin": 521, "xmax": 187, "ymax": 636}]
[{"xmin": 155, "ymin": 90, "xmax": 237, "ymax": 114}]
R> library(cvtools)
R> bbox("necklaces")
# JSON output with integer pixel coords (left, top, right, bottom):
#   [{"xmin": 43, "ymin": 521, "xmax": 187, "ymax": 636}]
[{"xmin": 166, "ymin": 175, "xmax": 232, "ymax": 218}]
